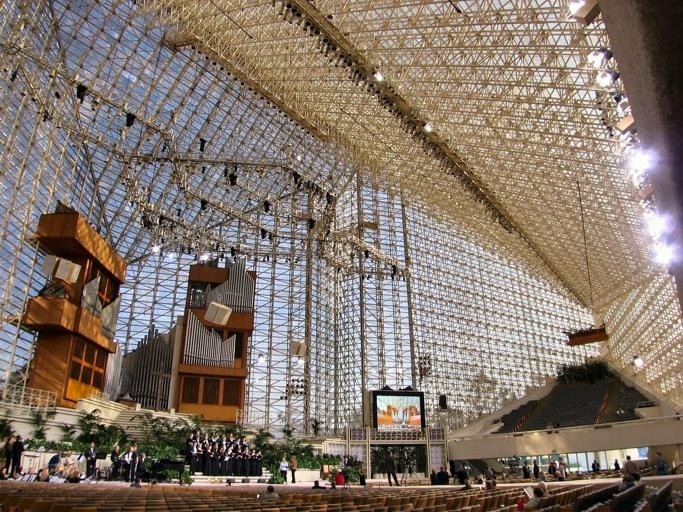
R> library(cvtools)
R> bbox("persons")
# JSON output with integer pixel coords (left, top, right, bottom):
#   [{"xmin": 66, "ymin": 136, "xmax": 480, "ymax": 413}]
[
  {"xmin": 591, "ymin": 460, "xmax": 601, "ymax": 471},
  {"xmin": 110, "ymin": 441, "xmax": 158, "ymax": 488},
  {"xmin": 84, "ymin": 441, "xmax": 96, "ymax": 480},
  {"xmin": 545, "ymin": 422, "xmax": 560, "ymax": 429},
  {"xmin": 616, "ymin": 408, "xmax": 624, "ymax": 414},
  {"xmin": 614, "ymin": 451, "xmax": 669, "ymax": 493},
  {"xmin": 278, "ymin": 456, "xmax": 289, "ymax": 482},
  {"xmin": 312, "ymin": 480, "xmax": 326, "ymax": 490},
  {"xmin": 257, "ymin": 486, "xmax": 279, "ymax": 500},
  {"xmin": 385, "ymin": 450, "xmax": 401, "ymax": 487},
  {"xmin": 329, "ymin": 482, "xmax": 337, "ymax": 489},
  {"xmin": 0, "ymin": 434, "xmax": 26, "ymax": 480},
  {"xmin": 430, "ymin": 457, "xmax": 570, "ymax": 512},
  {"xmin": 290, "ymin": 455, "xmax": 297, "ymax": 483},
  {"xmin": 32, "ymin": 451, "xmax": 85, "ymax": 482},
  {"xmin": 344, "ymin": 457, "xmax": 350, "ymax": 466},
  {"xmin": 184, "ymin": 428, "xmax": 263, "ymax": 476}
]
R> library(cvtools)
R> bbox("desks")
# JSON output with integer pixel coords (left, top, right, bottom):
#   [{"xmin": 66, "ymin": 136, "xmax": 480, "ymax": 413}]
[{"xmin": 20, "ymin": 451, "xmax": 87, "ymax": 475}]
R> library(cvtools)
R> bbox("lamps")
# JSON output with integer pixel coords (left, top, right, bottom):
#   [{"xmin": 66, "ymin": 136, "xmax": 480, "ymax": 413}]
[
  {"xmin": 568, "ymin": 0, "xmax": 673, "ymax": 267},
  {"xmin": 280, "ymin": 378, "xmax": 309, "ymax": 400}
]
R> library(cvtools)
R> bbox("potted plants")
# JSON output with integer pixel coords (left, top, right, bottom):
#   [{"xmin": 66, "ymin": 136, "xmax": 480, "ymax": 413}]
[{"xmin": 561, "ymin": 181, "xmax": 609, "ymax": 346}]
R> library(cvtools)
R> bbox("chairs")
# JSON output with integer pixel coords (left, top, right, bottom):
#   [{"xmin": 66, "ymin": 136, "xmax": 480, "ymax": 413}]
[{"xmin": 0, "ymin": 462, "xmax": 683, "ymax": 512}]
